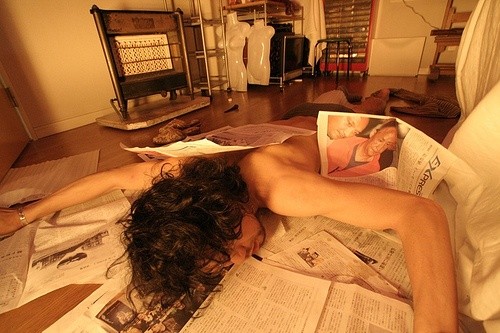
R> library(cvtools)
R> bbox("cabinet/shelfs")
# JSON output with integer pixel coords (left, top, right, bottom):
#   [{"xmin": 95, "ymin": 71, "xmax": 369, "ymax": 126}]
[
  {"xmin": 224, "ymin": 1, "xmax": 303, "ymax": 79},
  {"xmin": 163, "ymin": 0, "xmax": 232, "ymax": 103}
]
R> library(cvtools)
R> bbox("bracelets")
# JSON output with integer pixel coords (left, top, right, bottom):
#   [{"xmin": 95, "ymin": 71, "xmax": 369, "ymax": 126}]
[{"xmin": 17, "ymin": 205, "xmax": 29, "ymax": 226}]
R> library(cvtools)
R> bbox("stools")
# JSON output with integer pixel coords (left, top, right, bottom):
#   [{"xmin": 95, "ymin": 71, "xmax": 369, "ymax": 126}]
[{"xmin": 314, "ymin": 37, "xmax": 353, "ymax": 91}]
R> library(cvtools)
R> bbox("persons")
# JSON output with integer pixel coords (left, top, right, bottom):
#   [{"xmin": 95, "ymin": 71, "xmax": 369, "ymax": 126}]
[
  {"xmin": 137, "ymin": 282, "xmax": 208, "ymax": 333},
  {"xmin": 0, "ymin": 88, "xmax": 460, "ymax": 333},
  {"xmin": 215, "ymin": 12, "xmax": 275, "ymax": 92},
  {"xmin": 327, "ymin": 115, "xmax": 397, "ymax": 178}
]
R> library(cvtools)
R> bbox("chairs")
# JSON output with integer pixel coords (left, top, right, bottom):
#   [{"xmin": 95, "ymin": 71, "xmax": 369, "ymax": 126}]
[{"xmin": 429, "ymin": 0, "xmax": 473, "ymax": 80}]
